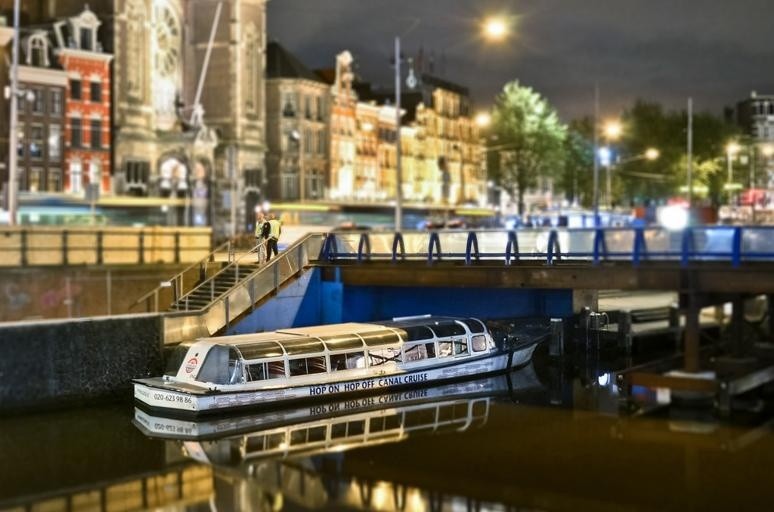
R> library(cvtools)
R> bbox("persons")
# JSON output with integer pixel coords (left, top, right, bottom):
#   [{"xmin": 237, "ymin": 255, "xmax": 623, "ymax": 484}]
[
  {"xmin": 259, "ymin": 213, "xmax": 282, "ymax": 262},
  {"xmin": 253, "ymin": 210, "xmax": 266, "ymax": 266}
]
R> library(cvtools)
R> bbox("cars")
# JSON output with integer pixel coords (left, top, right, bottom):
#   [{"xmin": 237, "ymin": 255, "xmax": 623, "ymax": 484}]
[{"xmin": 332, "ymin": 206, "xmax": 598, "ymax": 232}]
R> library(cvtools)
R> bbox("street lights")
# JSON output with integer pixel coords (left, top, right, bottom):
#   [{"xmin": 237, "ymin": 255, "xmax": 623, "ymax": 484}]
[
  {"xmin": 600, "ymin": 118, "xmax": 624, "ymax": 215},
  {"xmin": 725, "ymin": 141, "xmax": 743, "ymax": 206},
  {"xmin": 612, "ymin": 146, "xmax": 663, "ymax": 167},
  {"xmin": 0, "ymin": 86, "xmax": 37, "ymax": 222},
  {"xmin": 748, "ymin": 140, "xmax": 773, "ymax": 223},
  {"xmin": 390, "ymin": 34, "xmax": 418, "ymax": 253}
]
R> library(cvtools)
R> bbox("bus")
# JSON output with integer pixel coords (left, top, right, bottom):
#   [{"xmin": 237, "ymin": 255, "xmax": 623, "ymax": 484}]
[
  {"xmin": 14, "ymin": 192, "xmax": 207, "ymax": 228},
  {"xmin": 261, "ymin": 204, "xmax": 505, "ymax": 243}
]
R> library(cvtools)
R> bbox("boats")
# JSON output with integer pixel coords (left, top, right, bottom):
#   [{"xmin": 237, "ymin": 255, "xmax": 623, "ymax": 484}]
[
  {"xmin": 122, "ymin": 309, "xmax": 562, "ymax": 418},
  {"xmin": 125, "ymin": 359, "xmax": 551, "ymax": 474}
]
